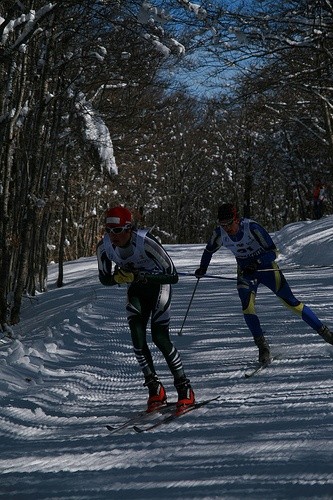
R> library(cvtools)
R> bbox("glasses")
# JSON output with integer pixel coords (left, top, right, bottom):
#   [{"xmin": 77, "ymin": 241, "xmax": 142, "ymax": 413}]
[
  {"xmin": 219, "ymin": 221, "xmax": 233, "ymax": 227},
  {"xmin": 104, "ymin": 224, "xmax": 130, "ymax": 234}
]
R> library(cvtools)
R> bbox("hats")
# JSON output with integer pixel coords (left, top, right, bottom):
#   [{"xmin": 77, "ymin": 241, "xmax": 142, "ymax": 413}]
[
  {"xmin": 218, "ymin": 203, "xmax": 236, "ymax": 224},
  {"xmin": 104, "ymin": 205, "xmax": 131, "ymax": 225}
]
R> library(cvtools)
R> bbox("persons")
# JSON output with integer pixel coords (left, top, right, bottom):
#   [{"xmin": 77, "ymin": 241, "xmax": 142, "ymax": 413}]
[
  {"xmin": 96, "ymin": 206, "xmax": 195, "ymax": 417},
  {"xmin": 312, "ymin": 184, "xmax": 326, "ymax": 220},
  {"xmin": 195, "ymin": 203, "xmax": 333, "ymax": 362}
]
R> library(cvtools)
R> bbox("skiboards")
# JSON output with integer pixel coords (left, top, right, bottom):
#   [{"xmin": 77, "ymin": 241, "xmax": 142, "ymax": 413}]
[
  {"xmin": 105, "ymin": 395, "xmax": 221, "ymax": 433},
  {"xmin": 243, "ymin": 354, "xmax": 281, "ymax": 379}
]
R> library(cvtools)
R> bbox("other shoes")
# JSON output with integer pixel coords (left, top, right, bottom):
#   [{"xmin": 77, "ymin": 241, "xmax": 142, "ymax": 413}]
[
  {"xmin": 147, "ymin": 382, "xmax": 168, "ymax": 411},
  {"xmin": 176, "ymin": 385, "xmax": 196, "ymax": 409},
  {"xmin": 318, "ymin": 327, "xmax": 333, "ymax": 346},
  {"xmin": 254, "ymin": 336, "xmax": 272, "ymax": 363}
]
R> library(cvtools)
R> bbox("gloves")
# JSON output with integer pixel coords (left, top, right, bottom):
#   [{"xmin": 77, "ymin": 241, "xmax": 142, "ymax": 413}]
[
  {"xmin": 244, "ymin": 263, "xmax": 258, "ymax": 275},
  {"xmin": 112, "ymin": 262, "xmax": 138, "ymax": 285},
  {"xmin": 194, "ymin": 268, "xmax": 206, "ymax": 278}
]
